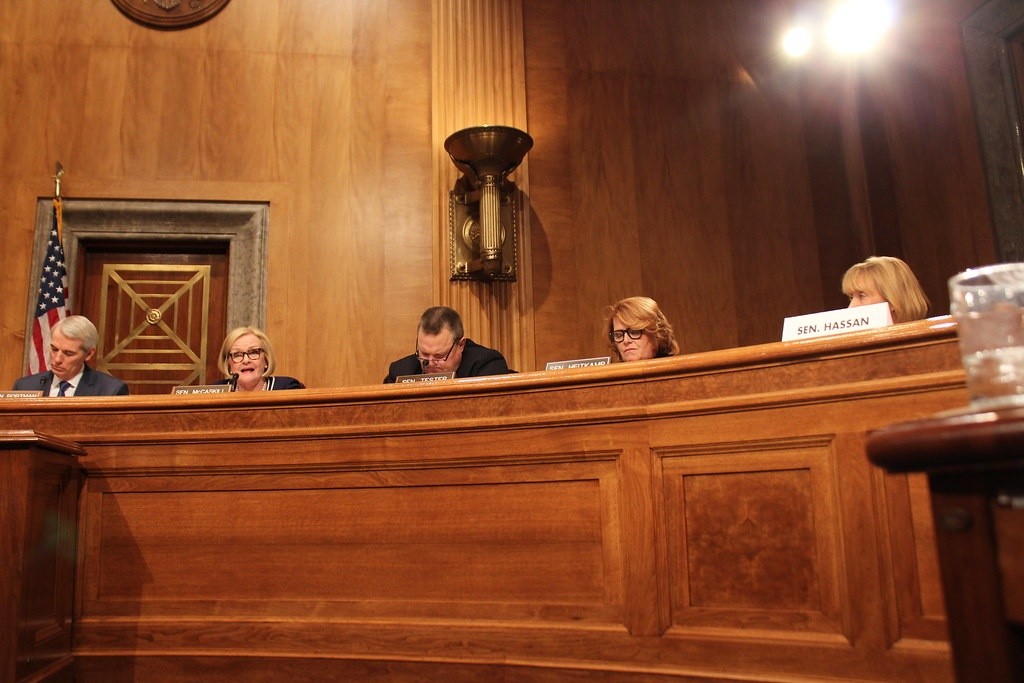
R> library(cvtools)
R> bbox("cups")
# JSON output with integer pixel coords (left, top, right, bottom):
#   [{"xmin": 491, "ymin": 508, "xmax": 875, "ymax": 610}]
[{"xmin": 947, "ymin": 262, "xmax": 1024, "ymax": 410}]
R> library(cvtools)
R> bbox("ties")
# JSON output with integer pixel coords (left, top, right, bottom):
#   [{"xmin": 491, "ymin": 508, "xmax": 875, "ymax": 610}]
[{"xmin": 56, "ymin": 381, "xmax": 70, "ymax": 397}]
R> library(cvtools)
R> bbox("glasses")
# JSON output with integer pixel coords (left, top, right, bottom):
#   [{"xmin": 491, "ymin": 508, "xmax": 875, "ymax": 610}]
[
  {"xmin": 415, "ymin": 337, "xmax": 462, "ymax": 361},
  {"xmin": 228, "ymin": 348, "xmax": 264, "ymax": 363},
  {"xmin": 609, "ymin": 327, "xmax": 642, "ymax": 343}
]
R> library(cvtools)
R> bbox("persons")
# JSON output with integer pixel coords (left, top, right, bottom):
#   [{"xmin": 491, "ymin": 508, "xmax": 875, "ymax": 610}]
[
  {"xmin": 842, "ymin": 256, "xmax": 929, "ymax": 324},
  {"xmin": 604, "ymin": 296, "xmax": 680, "ymax": 363},
  {"xmin": 14, "ymin": 316, "xmax": 128, "ymax": 396},
  {"xmin": 384, "ymin": 307, "xmax": 509, "ymax": 384},
  {"xmin": 214, "ymin": 326, "xmax": 304, "ymax": 391},
  {"xmin": 29, "ymin": 205, "xmax": 71, "ymax": 374}
]
